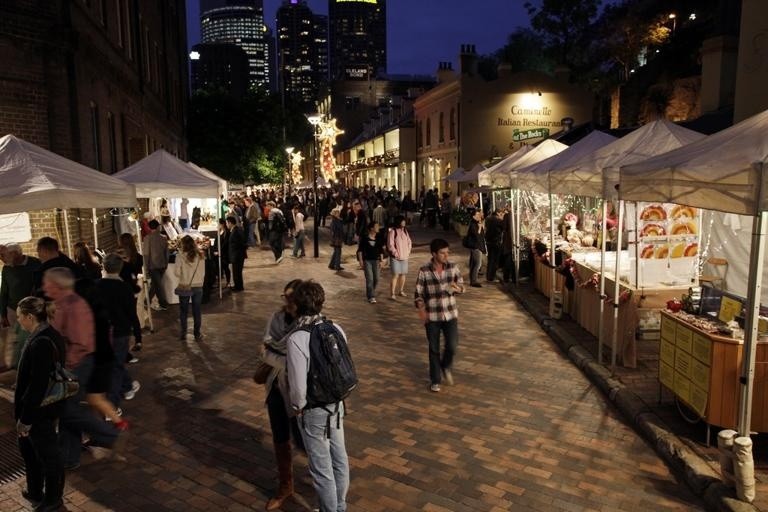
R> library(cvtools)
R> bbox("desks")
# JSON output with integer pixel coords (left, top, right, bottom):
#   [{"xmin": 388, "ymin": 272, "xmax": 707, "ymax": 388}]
[
  {"xmin": 653, "ymin": 303, "xmax": 766, "ymax": 447},
  {"xmin": 527, "ymin": 236, "xmax": 697, "ymax": 369}
]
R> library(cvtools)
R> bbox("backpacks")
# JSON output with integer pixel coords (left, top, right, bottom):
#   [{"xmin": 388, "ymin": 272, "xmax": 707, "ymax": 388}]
[
  {"xmin": 272, "ymin": 212, "xmax": 288, "ymax": 235},
  {"xmin": 287, "ymin": 316, "xmax": 360, "ymax": 409}
]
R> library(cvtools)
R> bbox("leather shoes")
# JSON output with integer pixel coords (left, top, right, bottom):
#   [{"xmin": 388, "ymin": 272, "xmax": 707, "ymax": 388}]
[
  {"xmin": 35, "ymin": 498, "xmax": 64, "ymax": 511},
  {"xmin": 22, "ymin": 486, "xmax": 43, "ymax": 501}
]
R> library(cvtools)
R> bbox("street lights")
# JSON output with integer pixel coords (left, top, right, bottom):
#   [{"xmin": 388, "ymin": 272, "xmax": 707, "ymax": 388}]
[
  {"xmin": 302, "ymin": 112, "xmax": 328, "ymax": 259},
  {"xmin": 668, "ymin": 13, "xmax": 676, "ymax": 31},
  {"xmin": 284, "ymin": 145, "xmax": 293, "ymax": 195}
]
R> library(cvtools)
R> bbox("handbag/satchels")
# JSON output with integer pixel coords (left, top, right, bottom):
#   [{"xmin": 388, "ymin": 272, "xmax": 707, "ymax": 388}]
[
  {"xmin": 253, "ymin": 361, "xmax": 271, "ymax": 383},
  {"xmin": 40, "ymin": 367, "xmax": 81, "ymax": 407},
  {"xmin": 174, "ymin": 283, "xmax": 195, "ymax": 297}
]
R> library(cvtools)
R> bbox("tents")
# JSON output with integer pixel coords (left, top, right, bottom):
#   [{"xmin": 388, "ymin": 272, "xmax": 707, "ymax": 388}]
[
  {"xmin": 113, "ymin": 149, "xmax": 227, "ymax": 299},
  {"xmin": 0, "ymin": 135, "xmax": 154, "ymax": 332}
]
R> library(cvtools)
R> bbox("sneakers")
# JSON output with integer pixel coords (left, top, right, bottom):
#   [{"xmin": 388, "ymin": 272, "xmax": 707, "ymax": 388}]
[
  {"xmin": 124, "ymin": 380, "xmax": 140, "ymax": 399},
  {"xmin": 430, "ymin": 384, "xmax": 441, "ymax": 393},
  {"xmin": 370, "ymin": 297, "xmax": 377, "ymax": 304}
]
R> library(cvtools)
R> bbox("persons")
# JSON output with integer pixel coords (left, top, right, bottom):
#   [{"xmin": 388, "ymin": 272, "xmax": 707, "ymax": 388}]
[
  {"xmin": 258, "ymin": 279, "xmax": 350, "ymax": 511},
  {"xmin": 414, "ymin": 238, "xmax": 466, "ymax": 391},
  {"xmin": 593, "ymin": 201, "xmax": 618, "ymax": 251},
  {"xmin": 1, "ymin": 232, "xmax": 144, "ymax": 512},
  {"xmin": 224, "ymin": 186, "xmax": 512, "ymax": 303},
  {"xmin": 143, "ymin": 195, "xmax": 248, "ymax": 340}
]
